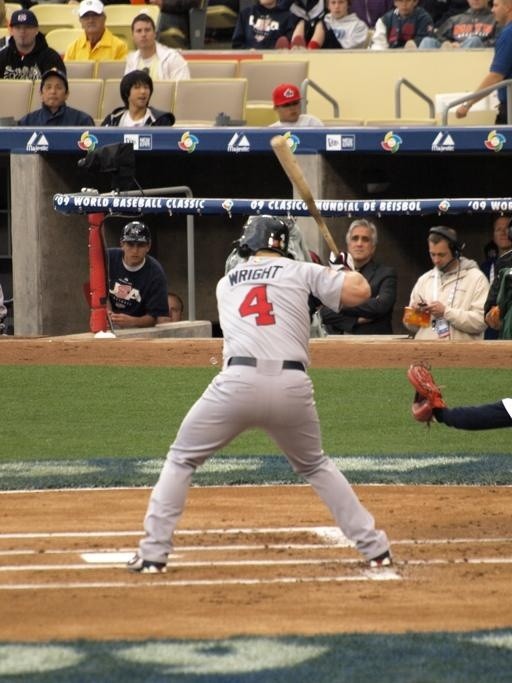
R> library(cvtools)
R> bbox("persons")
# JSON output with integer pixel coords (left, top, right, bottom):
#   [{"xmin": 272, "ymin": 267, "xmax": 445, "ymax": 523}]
[
  {"xmin": 104, "ymin": 219, "xmax": 170, "ymax": 331},
  {"xmin": 479, "ymin": 239, "xmax": 499, "ymax": 285},
  {"xmin": 230, "ymin": 0, "xmax": 502, "ymax": 50},
  {"xmin": 267, "ymin": 83, "xmax": 324, "ymax": 128},
  {"xmin": 320, "ymin": 217, "xmax": 399, "ymax": 335},
  {"xmin": 405, "ymin": 361, "xmax": 511, "ymax": 431},
  {"xmin": 126, "ymin": 213, "xmax": 393, "ymax": 577},
  {"xmin": 482, "ymin": 254, "xmax": 511, "ymax": 340},
  {"xmin": 455, "ymin": 2, "xmax": 511, "ymax": 124},
  {"xmin": 124, "ymin": 14, "xmax": 192, "ymax": 80},
  {"xmin": 403, "ymin": 223, "xmax": 493, "ymax": 342},
  {"xmin": 165, "ymin": 292, "xmax": 184, "ymax": 321},
  {"xmin": 100, "ymin": 69, "xmax": 176, "ymax": 127},
  {"xmin": 62, "ymin": 1, "xmax": 128, "ymax": 63},
  {"xmin": 16, "ymin": 67, "xmax": 95, "ymax": 126},
  {"xmin": 492, "ymin": 213, "xmax": 512, "ymax": 280},
  {"xmin": 0, "ymin": 1, "xmax": 67, "ymax": 80}
]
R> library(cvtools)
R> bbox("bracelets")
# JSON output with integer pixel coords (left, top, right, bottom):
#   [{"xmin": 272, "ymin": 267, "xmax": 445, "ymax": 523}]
[{"xmin": 463, "ymin": 101, "xmax": 471, "ymax": 109}]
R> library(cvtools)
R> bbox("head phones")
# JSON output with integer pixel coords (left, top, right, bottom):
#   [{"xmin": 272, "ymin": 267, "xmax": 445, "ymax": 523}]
[{"xmin": 430, "ymin": 227, "xmax": 466, "ymax": 258}]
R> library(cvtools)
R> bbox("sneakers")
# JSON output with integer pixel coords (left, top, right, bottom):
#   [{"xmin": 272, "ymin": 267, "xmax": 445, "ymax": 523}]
[
  {"xmin": 366, "ymin": 549, "xmax": 393, "ymax": 567},
  {"xmin": 126, "ymin": 552, "xmax": 168, "ymax": 574},
  {"xmin": 275, "ymin": 36, "xmax": 289, "ymax": 50},
  {"xmin": 290, "ymin": 34, "xmax": 307, "ymax": 51}
]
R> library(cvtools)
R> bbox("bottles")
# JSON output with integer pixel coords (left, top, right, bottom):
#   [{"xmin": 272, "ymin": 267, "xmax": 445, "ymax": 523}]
[{"xmin": 435, "ymin": 317, "xmax": 449, "ymax": 341}]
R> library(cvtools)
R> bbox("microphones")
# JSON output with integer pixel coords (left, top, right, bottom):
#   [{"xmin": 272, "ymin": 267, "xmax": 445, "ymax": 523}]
[{"xmin": 439, "ymin": 255, "xmax": 461, "ymax": 270}]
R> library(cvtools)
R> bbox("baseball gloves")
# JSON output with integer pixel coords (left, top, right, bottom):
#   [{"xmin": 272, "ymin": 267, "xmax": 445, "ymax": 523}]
[{"xmin": 408, "ymin": 363, "xmax": 447, "ymax": 421}]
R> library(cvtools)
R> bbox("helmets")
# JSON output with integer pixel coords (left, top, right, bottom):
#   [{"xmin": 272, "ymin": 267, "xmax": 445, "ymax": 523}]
[
  {"xmin": 121, "ymin": 220, "xmax": 151, "ymax": 243},
  {"xmin": 233, "ymin": 216, "xmax": 289, "ymax": 257}
]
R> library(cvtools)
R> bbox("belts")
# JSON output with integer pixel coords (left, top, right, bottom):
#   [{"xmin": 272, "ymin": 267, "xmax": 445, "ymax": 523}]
[{"xmin": 227, "ymin": 356, "xmax": 306, "ymax": 371}]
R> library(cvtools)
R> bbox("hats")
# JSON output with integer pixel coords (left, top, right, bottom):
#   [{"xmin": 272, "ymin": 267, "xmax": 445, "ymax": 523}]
[
  {"xmin": 10, "ymin": 10, "xmax": 39, "ymax": 26},
  {"xmin": 272, "ymin": 82, "xmax": 304, "ymax": 108},
  {"xmin": 41, "ymin": 67, "xmax": 66, "ymax": 82},
  {"xmin": 79, "ymin": 0, "xmax": 104, "ymax": 16}
]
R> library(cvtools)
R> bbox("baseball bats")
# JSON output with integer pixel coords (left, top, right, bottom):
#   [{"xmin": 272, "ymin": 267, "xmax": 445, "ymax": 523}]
[{"xmin": 271, "ymin": 135, "xmax": 351, "ymax": 270}]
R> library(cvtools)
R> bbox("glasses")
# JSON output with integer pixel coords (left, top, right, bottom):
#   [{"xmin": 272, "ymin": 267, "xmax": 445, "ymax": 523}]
[{"xmin": 281, "ymin": 99, "xmax": 299, "ymax": 106}]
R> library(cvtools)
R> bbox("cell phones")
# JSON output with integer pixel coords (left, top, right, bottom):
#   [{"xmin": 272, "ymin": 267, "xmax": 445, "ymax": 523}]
[{"xmin": 418, "ymin": 303, "xmax": 427, "ymax": 306}]
